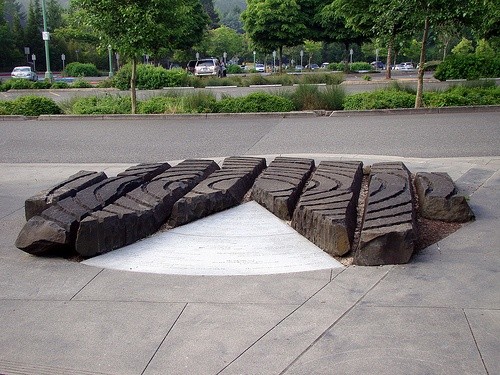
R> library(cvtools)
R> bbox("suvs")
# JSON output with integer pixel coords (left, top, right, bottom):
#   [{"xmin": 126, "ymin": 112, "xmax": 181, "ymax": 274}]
[
  {"xmin": 370, "ymin": 61, "xmax": 383, "ymax": 69},
  {"xmin": 399, "ymin": 61, "xmax": 413, "ymax": 69},
  {"xmin": 194, "ymin": 58, "xmax": 222, "ymax": 78},
  {"xmin": 185, "ymin": 60, "xmax": 197, "ymax": 74}
]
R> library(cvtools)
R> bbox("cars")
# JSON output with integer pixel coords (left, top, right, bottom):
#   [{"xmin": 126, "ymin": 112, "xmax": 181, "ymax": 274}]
[
  {"xmin": 304, "ymin": 64, "xmax": 319, "ymax": 69},
  {"xmin": 10, "ymin": 66, "xmax": 39, "ymax": 82},
  {"xmin": 294, "ymin": 65, "xmax": 304, "ymax": 70}
]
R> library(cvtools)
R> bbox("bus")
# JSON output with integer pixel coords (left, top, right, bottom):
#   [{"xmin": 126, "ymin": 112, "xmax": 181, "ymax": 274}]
[{"xmin": 254, "ymin": 64, "xmax": 265, "ymax": 72}]
